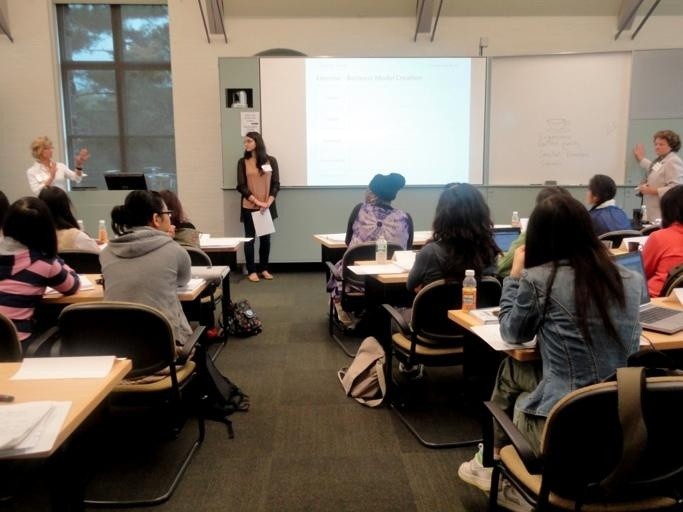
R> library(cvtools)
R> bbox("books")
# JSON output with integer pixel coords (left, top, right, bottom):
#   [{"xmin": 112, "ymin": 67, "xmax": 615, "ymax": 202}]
[
  {"xmin": 198, "ymin": 232, "xmax": 210, "ymax": 248},
  {"xmin": 0, "ymin": 400, "xmax": 73, "ymax": 457},
  {"xmin": 318, "ymin": 232, "xmax": 349, "ymax": 242},
  {"xmin": 43, "ymin": 274, "xmax": 94, "ymax": 296},
  {"xmin": 177, "ymin": 276, "xmax": 206, "ymax": 295},
  {"xmin": 468, "ymin": 305, "xmax": 505, "ymax": 325},
  {"xmin": 392, "ymin": 250, "xmax": 416, "ymax": 272}
]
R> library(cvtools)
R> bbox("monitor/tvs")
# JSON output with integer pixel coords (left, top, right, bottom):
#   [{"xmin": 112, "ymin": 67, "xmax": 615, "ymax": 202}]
[
  {"xmin": 104, "ymin": 172, "xmax": 148, "ymax": 190},
  {"xmin": 492, "ymin": 226, "xmax": 522, "ymax": 252}
]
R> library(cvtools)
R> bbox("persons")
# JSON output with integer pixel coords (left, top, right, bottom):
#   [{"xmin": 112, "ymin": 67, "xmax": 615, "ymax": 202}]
[
  {"xmin": 39, "ymin": 186, "xmax": 99, "ymax": 252},
  {"xmin": 325, "ymin": 173, "xmax": 414, "ymax": 303},
  {"xmin": 26, "ymin": 135, "xmax": 91, "ymax": 200},
  {"xmin": 0, "ymin": 192, "xmax": 80, "ymax": 354},
  {"xmin": 642, "ymin": 184, "xmax": 683, "ymax": 298},
  {"xmin": 159, "ymin": 189, "xmax": 200, "ymax": 249},
  {"xmin": 98, "ymin": 189, "xmax": 216, "ymax": 367},
  {"xmin": 455, "ymin": 194, "xmax": 643, "ymax": 512},
  {"xmin": 236, "ymin": 131, "xmax": 280, "ymax": 282},
  {"xmin": 496, "ymin": 187, "xmax": 572, "ymax": 277},
  {"xmin": 584, "ymin": 173, "xmax": 633, "ymax": 248},
  {"xmin": 391, "ymin": 181, "xmax": 504, "ymax": 387},
  {"xmin": 633, "ymin": 129, "xmax": 683, "ymax": 224}
]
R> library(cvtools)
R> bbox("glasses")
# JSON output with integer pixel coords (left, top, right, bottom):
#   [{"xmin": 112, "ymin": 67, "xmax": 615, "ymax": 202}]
[{"xmin": 159, "ymin": 210, "xmax": 174, "ymax": 217}]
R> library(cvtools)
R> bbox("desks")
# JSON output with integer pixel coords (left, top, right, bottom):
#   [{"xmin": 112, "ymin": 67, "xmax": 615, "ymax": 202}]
[
  {"xmin": 447, "ymin": 296, "xmax": 683, "ymax": 362},
  {"xmin": 312, "ymin": 231, "xmax": 435, "ymax": 279},
  {"xmin": 99, "ymin": 237, "xmax": 241, "ymax": 302},
  {"xmin": 42, "ymin": 265, "xmax": 231, "ymax": 362},
  {"xmin": 0, "ymin": 356, "xmax": 133, "ymax": 462},
  {"xmin": 354, "ymin": 248, "xmax": 629, "ymax": 358}
]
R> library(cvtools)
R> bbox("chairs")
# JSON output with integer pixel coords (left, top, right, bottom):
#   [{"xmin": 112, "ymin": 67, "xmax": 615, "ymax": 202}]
[
  {"xmin": 182, "ymin": 246, "xmax": 222, "ymax": 310},
  {"xmin": 641, "ymin": 225, "xmax": 661, "ymax": 236},
  {"xmin": 659, "ymin": 265, "xmax": 683, "ymax": 297},
  {"xmin": 379, "ymin": 275, "xmax": 502, "ymax": 448},
  {"xmin": 59, "ymin": 250, "xmax": 100, "ymax": 274},
  {"xmin": 0, "ymin": 307, "xmax": 57, "ymax": 360},
  {"xmin": 328, "ymin": 242, "xmax": 405, "ymax": 359},
  {"xmin": 56, "ymin": 301, "xmax": 206, "ymax": 508},
  {"xmin": 598, "ymin": 229, "xmax": 643, "ymax": 248},
  {"xmin": 482, "ymin": 377, "xmax": 683, "ymax": 512}
]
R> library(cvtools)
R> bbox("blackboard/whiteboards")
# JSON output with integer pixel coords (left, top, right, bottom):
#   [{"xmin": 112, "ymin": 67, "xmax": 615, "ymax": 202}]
[
  {"xmin": 491, "ymin": 49, "xmax": 632, "ymax": 186},
  {"xmin": 260, "ymin": 56, "xmax": 487, "ymax": 187}
]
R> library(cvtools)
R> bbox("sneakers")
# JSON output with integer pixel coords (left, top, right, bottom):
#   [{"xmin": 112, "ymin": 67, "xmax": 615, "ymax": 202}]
[
  {"xmin": 457, "ymin": 452, "xmax": 502, "ymax": 492},
  {"xmin": 484, "ymin": 480, "xmax": 536, "ymax": 512}
]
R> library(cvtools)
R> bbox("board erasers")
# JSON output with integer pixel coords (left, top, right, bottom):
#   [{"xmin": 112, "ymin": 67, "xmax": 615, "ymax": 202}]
[
  {"xmin": 530, "ymin": 183, "xmax": 542, "ymax": 186},
  {"xmin": 544, "ymin": 180, "xmax": 557, "ymax": 185}
]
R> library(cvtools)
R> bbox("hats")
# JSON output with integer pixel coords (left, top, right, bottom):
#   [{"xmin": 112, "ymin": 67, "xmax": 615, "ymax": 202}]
[{"xmin": 369, "ymin": 173, "xmax": 405, "ymax": 201}]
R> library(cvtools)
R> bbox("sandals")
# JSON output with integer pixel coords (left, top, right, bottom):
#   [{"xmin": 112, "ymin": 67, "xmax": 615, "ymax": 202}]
[
  {"xmin": 248, "ymin": 273, "xmax": 260, "ymax": 282},
  {"xmin": 261, "ymin": 271, "xmax": 274, "ymax": 279}
]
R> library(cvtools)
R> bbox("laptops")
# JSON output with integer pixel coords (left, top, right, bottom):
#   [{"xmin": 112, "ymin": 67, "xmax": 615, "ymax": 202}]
[{"xmin": 611, "ymin": 251, "xmax": 683, "ymax": 335}]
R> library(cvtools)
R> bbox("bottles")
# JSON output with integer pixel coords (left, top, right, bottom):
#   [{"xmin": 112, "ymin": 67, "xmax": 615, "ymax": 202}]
[
  {"xmin": 77, "ymin": 219, "xmax": 85, "ymax": 232},
  {"xmin": 98, "ymin": 220, "xmax": 108, "ymax": 241},
  {"xmin": 462, "ymin": 271, "xmax": 477, "ymax": 313},
  {"xmin": 512, "ymin": 210, "xmax": 520, "ymax": 226},
  {"xmin": 641, "ymin": 205, "xmax": 647, "ymax": 220},
  {"xmin": 376, "ymin": 236, "xmax": 388, "ymax": 264}
]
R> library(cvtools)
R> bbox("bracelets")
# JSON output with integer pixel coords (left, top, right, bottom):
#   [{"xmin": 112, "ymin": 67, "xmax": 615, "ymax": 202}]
[
  {"xmin": 253, "ymin": 198, "xmax": 256, "ymax": 202},
  {"xmin": 76, "ymin": 167, "xmax": 82, "ymax": 171}
]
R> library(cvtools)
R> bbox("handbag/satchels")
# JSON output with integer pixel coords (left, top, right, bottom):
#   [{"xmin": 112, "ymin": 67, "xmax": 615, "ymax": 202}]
[
  {"xmin": 200, "ymin": 348, "xmax": 250, "ymax": 440},
  {"xmin": 218, "ymin": 299, "xmax": 265, "ymax": 336},
  {"xmin": 336, "ymin": 335, "xmax": 386, "ymax": 407}
]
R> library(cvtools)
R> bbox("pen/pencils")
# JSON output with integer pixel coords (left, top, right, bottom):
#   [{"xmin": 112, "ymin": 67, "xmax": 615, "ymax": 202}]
[{"xmin": 0, "ymin": 392, "xmax": 14, "ymax": 404}]
